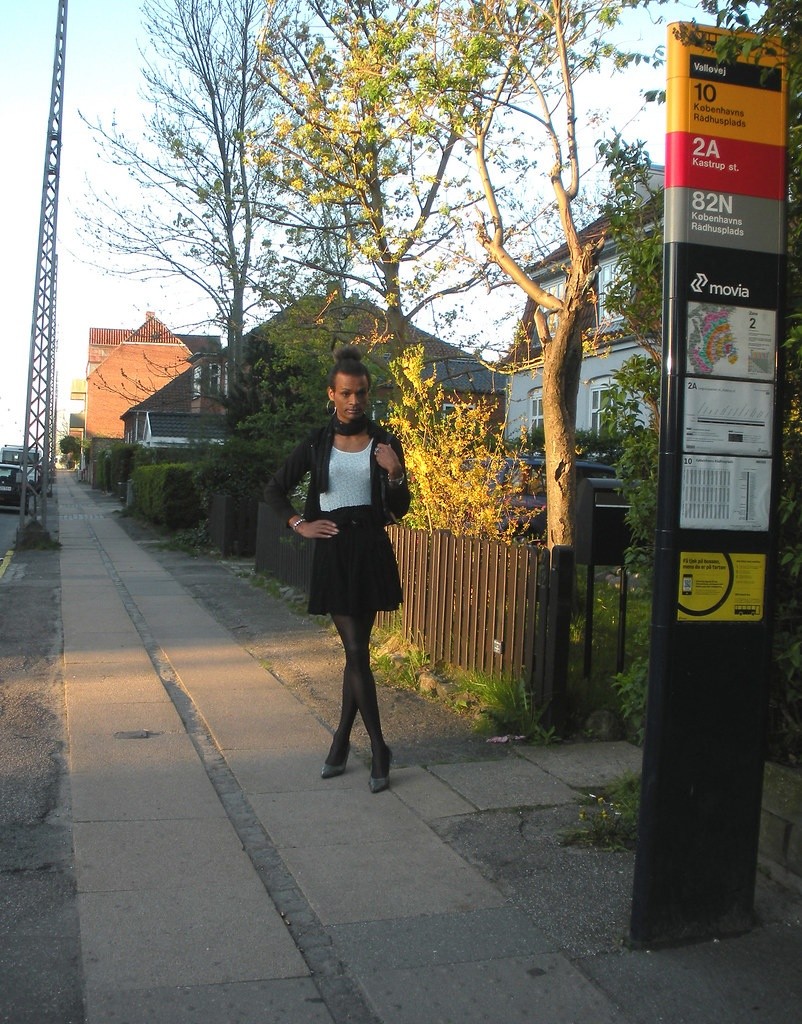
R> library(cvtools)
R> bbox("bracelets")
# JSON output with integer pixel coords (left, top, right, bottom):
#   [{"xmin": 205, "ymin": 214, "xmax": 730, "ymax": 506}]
[
  {"xmin": 293, "ymin": 519, "xmax": 307, "ymax": 532},
  {"xmin": 387, "ymin": 472, "xmax": 404, "ymax": 486}
]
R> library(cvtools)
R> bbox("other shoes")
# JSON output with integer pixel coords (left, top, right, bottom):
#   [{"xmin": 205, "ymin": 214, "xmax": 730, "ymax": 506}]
[
  {"xmin": 321, "ymin": 744, "xmax": 351, "ymax": 777},
  {"xmin": 368, "ymin": 747, "xmax": 393, "ymax": 792}
]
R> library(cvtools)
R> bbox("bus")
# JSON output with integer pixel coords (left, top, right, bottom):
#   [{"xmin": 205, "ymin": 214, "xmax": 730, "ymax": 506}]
[{"xmin": 0, "ymin": 445, "xmax": 40, "ymax": 489}]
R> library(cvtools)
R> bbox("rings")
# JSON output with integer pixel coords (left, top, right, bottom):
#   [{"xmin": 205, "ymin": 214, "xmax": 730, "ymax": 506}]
[
  {"xmin": 375, "ymin": 447, "xmax": 381, "ymax": 452},
  {"xmin": 374, "ymin": 451, "xmax": 377, "ymax": 456}
]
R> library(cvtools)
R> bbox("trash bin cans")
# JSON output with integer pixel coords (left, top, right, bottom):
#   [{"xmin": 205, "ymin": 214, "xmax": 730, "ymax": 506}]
[{"xmin": 78, "ymin": 470, "xmax": 83, "ymax": 481}]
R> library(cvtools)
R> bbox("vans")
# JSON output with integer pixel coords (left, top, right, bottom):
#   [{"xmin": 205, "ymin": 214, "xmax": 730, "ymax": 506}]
[{"xmin": 461, "ymin": 458, "xmax": 618, "ymax": 547}]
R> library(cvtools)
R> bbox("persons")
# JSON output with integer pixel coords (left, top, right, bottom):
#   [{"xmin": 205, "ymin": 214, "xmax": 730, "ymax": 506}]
[{"xmin": 263, "ymin": 344, "xmax": 411, "ymax": 793}]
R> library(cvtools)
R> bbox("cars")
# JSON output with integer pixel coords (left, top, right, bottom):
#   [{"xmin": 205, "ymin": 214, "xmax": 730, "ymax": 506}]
[{"xmin": 0, "ymin": 464, "xmax": 29, "ymax": 514}]
